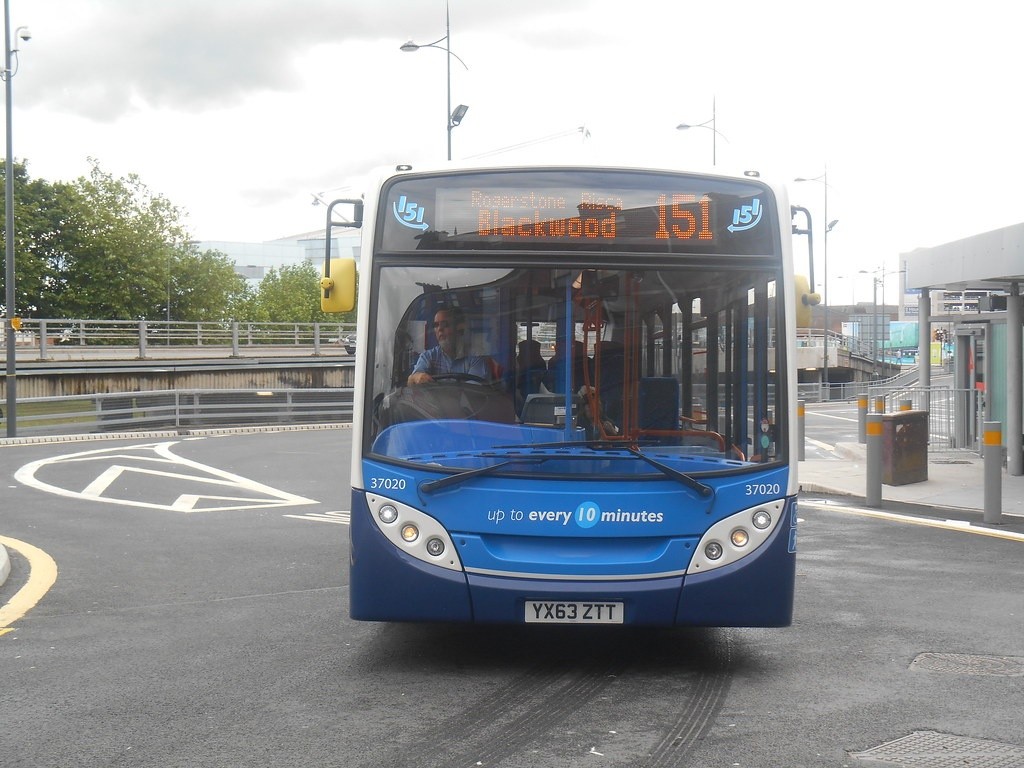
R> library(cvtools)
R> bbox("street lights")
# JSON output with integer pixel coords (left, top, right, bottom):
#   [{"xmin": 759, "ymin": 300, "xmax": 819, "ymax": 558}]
[
  {"xmin": 166, "ymin": 240, "xmax": 200, "ymax": 344},
  {"xmin": 858, "ymin": 266, "xmax": 906, "ymax": 382},
  {"xmin": 794, "ymin": 165, "xmax": 829, "ymax": 383},
  {"xmin": 677, "ymin": 95, "xmax": 724, "ymax": 165},
  {"xmin": 398, "ymin": 0, "xmax": 471, "ymax": 165},
  {"xmin": 0, "ymin": 0, "xmax": 33, "ymax": 437}
]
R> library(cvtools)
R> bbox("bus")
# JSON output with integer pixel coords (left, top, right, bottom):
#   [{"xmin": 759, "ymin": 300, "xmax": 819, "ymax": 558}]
[{"xmin": 321, "ymin": 166, "xmax": 821, "ymax": 631}]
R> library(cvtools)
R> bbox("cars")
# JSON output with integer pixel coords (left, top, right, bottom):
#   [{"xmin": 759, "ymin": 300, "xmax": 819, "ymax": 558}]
[{"xmin": 344, "ymin": 334, "xmax": 357, "ymax": 354}]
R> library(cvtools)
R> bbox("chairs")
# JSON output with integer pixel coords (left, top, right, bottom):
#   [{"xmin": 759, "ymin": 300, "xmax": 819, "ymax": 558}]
[{"xmin": 516, "ymin": 350, "xmax": 678, "ymax": 445}]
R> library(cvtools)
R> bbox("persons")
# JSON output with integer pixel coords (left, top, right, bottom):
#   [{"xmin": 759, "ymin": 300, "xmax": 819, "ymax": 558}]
[
  {"xmin": 407, "ymin": 308, "xmax": 491, "ymax": 388},
  {"xmin": 516, "ymin": 339, "xmax": 593, "ymax": 406},
  {"xmin": 896, "ymin": 348, "xmax": 902, "ymax": 365}
]
